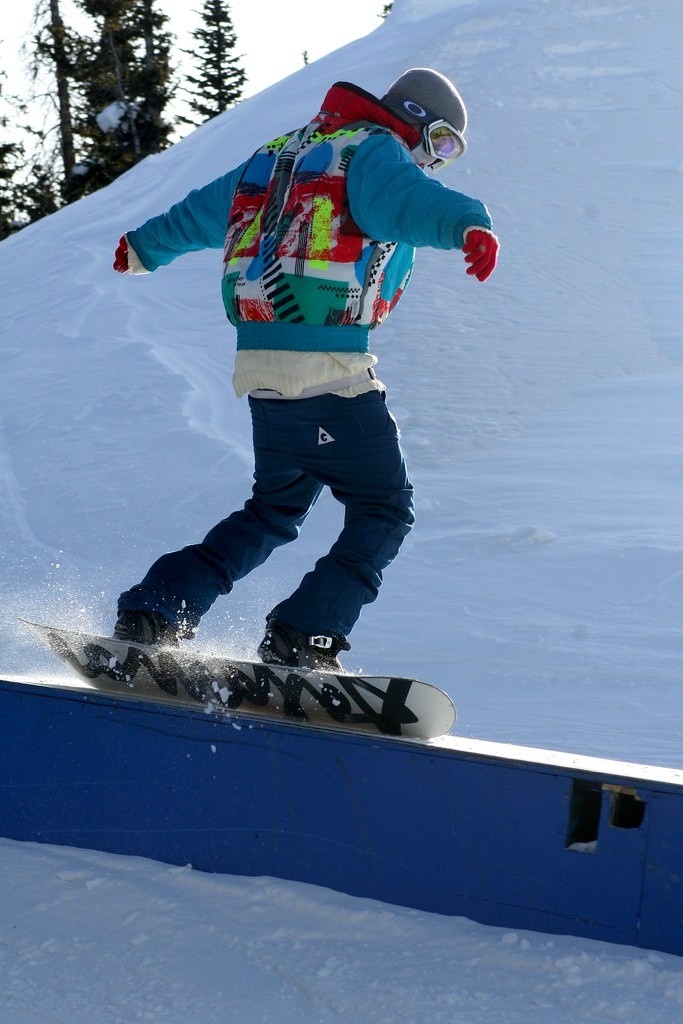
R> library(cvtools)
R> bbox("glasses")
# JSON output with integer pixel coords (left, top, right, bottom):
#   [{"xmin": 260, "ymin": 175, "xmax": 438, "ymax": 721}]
[{"xmin": 423, "ymin": 117, "xmax": 466, "ymax": 170}]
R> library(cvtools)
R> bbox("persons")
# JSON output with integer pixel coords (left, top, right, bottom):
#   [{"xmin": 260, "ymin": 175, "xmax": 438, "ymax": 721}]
[{"xmin": 114, "ymin": 69, "xmax": 499, "ymax": 670}]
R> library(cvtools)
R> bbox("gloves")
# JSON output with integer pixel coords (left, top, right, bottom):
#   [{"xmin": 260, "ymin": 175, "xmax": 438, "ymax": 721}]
[
  {"xmin": 463, "ymin": 229, "xmax": 498, "ymax": 281},
  {"xmin": 113, "ymin": 235, "xmax": 129, "ymax": 274}
]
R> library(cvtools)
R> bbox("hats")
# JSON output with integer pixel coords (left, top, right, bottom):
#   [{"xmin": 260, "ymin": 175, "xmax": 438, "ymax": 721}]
[{"xmin": 384, "ymin": 66, "xmax": 466, "ymax": 135}]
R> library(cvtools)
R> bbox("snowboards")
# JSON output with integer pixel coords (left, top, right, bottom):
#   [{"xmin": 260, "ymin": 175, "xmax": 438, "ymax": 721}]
[{"xmin": 13, "ymin": 614, "xmax": 455, "ymax": 743}]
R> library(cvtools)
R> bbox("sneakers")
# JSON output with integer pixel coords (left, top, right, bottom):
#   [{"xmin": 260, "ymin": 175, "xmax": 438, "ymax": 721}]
[
  {"xmin": 260, "ymin": 630, "xmax": 344, "ymax": 672},
  {"xmin": 112, "ymin": 613, "xmax": 181, "ymax": 650}
]
